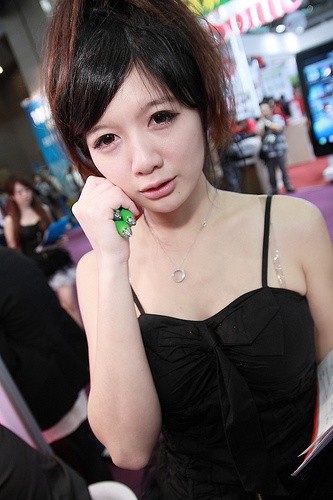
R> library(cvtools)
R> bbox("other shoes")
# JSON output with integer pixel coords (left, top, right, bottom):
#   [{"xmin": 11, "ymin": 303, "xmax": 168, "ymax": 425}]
[
  {"xmin": 287, "ymin": 188, "xmax": 292, "ymax": 192},
  {"xmin": 272, "ymin": 190, "xmax": 278, "ymax": 195}
]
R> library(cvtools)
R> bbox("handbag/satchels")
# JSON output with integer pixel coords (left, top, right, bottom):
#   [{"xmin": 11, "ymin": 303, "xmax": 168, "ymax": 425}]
[{"xmin": 259, "ymin": 148, "xmax": 265, "ymax": 159}]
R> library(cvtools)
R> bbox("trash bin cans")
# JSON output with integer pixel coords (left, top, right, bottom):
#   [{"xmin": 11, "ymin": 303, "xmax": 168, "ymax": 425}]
[{"xmin": 229, "ymin": 135, "xmax": 284, "ymax": 196}]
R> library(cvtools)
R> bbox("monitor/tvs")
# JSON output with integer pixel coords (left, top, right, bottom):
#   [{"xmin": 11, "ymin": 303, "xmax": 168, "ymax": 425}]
[{"xmin": 295, "ymin": 38, "xmax": 333, "ymax": 157}]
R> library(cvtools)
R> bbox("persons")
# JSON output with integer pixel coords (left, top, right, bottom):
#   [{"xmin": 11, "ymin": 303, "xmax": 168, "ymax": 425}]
[
  {"xmin": 44, "ymin": 1, "xmax": 332, "ymax": 500},
  {"xmin": 215, "ymin": 131, "xmax": 243, "ymax": 193},
  {"xmin": 292, "ymin": 82, "xmax": 307, "ymax": 123},
  {"xmin": 263, "ymin": 95, "xmax": 291, "ymax": 126},
  {"xmin": 0, "ymin": 166, "xmax": 105, "ymax": 500},
  {"xmin": 255, "ymin": 99, "xmax": 296, "ymax": 195}
]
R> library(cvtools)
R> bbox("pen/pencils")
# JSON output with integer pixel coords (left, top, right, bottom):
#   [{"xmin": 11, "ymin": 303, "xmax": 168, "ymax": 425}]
[
  {"xmin": 115, "ymin": 221, "xmax": 133, "ymax": 239},
  {"xmin": 113, "ymin": 209, "xmax": 122, "ymax": 221},
  {"xmin": 120, "ymin": 208, "xmax": 136, "ymax": 226}
]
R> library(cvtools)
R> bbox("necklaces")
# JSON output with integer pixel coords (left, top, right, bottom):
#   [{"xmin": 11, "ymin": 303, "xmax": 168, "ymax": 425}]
[{"xmin": 142, "ymin": 189, "xmax": 218, "ymax": 282}]
